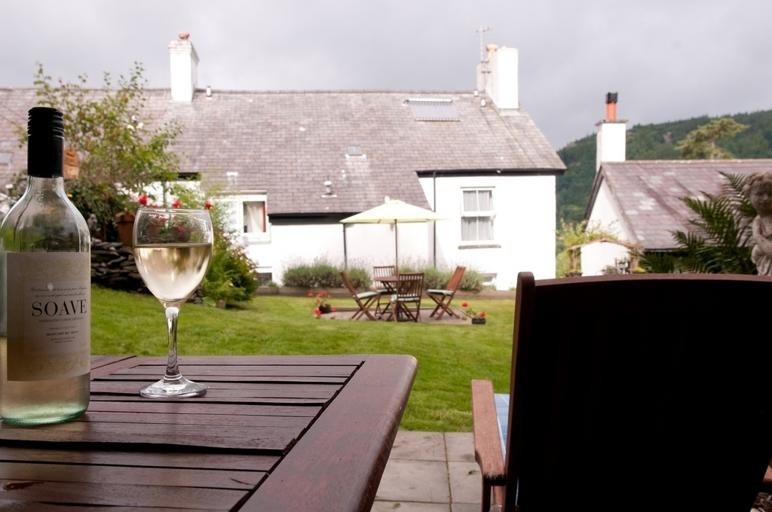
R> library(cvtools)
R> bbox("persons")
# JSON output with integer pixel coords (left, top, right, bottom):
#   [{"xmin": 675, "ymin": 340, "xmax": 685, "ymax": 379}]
[{"xmin": 742, "ymin": 171, "xmax": 771, "ymax": 275}]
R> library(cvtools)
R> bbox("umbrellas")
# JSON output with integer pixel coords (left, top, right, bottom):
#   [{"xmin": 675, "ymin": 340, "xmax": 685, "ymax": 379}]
[{"xmin": 338, "ymin": 197, "xmax": 447, "ymax": 321}]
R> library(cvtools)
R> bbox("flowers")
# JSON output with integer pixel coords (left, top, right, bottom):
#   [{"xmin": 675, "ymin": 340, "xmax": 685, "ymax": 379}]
[
  {"xmin": 459, "ymin": 301, "xmax": 487, "ymax": 321},
  {"xmin": 306, "ymin": 290, "xmax": 338, "ymax": 320}
]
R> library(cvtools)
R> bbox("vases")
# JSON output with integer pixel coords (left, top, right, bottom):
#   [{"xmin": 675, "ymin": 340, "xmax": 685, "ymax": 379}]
[
  {"xmin": 319, "ymin": 312, "xmax": 337, "ymax": 322},
  {"xmin": 472, "ymin": 318, "xmax": 486, "ymax": 325}
]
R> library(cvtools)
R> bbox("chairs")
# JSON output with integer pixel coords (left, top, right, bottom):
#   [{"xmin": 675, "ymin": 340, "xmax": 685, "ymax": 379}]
[
  {"xmin": 338, "ymin": 265, "xmax": 467, "ymax": 323},
  {"xmin": 470, "ymin": 271, "xmax": 772, "ymax": 512}
]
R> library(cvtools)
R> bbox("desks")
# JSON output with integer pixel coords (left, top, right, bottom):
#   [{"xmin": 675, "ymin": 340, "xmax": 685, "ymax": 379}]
[{"xmin": 1, "ymin": 353, "xmax": 417, "ymax": 512}]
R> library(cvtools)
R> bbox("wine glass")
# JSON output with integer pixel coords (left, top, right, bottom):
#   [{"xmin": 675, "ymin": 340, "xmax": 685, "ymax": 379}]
[{"xmin": 131, "ymin": 206, "xmax": 215, "ymax": 399}]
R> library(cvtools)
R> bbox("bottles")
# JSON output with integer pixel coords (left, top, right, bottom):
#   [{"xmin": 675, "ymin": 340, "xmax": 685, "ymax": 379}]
[{"xmin": 0, "ymin": 106, "xmax": 91, "ymax": 428}]
